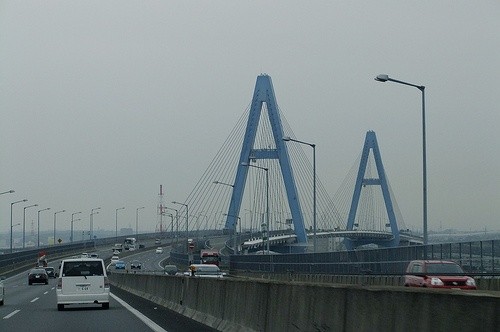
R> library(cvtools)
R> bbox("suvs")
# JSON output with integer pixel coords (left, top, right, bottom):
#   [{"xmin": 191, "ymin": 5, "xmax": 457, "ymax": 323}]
[
  {"xmin": 406, "ymin": 258, "xmax": 477, "ymax": 291},
  {"xmin": 56, "ymin": 258, "xmax": 110, "ymax": 311}
]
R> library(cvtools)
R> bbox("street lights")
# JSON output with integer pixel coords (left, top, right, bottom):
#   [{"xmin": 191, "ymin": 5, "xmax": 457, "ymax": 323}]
[
  {"xmin": 281, "ymin": 136, "xmax": 318, "ymax": 252},
  {"xmin": 135, "ymin": 207, "xmax": 145, "ymax": 235},
  {"xmin": 375, "ymin": 74, "xmax": 428, "ymax": 260},
  {"xmin": 10, "ymin": 199, "xmax": 28, "ymax": 254},
  {"xmin": 222, "ymin": 213, "xmax": 242, "ymax": 255},
  {"xmin": 37, "ymin": 208, "xmax": 50, "ymax": 247},
  {"xmin": 171, "ymin": 201, "xmax": 189, "ymax": 253},
  {"xmin": 115, "ymin": 207, "xmax": 125, "ymax": 238},
  {"xmin": 22, "ymin": 204, "xmax": 38, "ymax": 248},
  {"xmin": 90, "ymin": 207, "xmax": 101, "ymax": 240},
  {"xmin": 70, "ymin": 211, "xmax": 82, "ymax": 243},
  {"xmin": 241, "ymin": 163, "xmax": 269, "ymax": 255},
  {"xmin": 54, "ymin": 209, "xmax": 66, "ymax": 244}
]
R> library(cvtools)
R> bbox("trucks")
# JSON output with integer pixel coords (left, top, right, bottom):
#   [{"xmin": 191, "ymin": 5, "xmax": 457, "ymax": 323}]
[{"xmin": 187, "ymin": 237, "xmax": 222, "ymax": 268}]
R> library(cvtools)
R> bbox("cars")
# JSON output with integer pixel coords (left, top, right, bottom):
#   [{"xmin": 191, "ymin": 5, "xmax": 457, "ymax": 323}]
[
  {"xmin": 131, "ymin": 261, "xmax": 143, "ymax": 270},
  {"xmin": 45, "ymin": 267, "xmax": 57, "ymax": 279},
  {"xmin": 72, "ymin": 237, "xmax": 145, "ymax": 266},
  {"xmin": 155, "ymin": 239, "xmax": 163, "ymax": 255},
  {"xmin": 114, "ymin": 262, "xmax": 125, "ymax": 270},
  {"xmin": 0, "ymin": 276, "xmax": 6, "ymax": 305},
  {"xmin": 28, "ymin": 269, "xmax": 49, "ymax": 285},
  {"xmin": 185, "ymin": 263, "xmax": 229, "ymax": 281}
]
